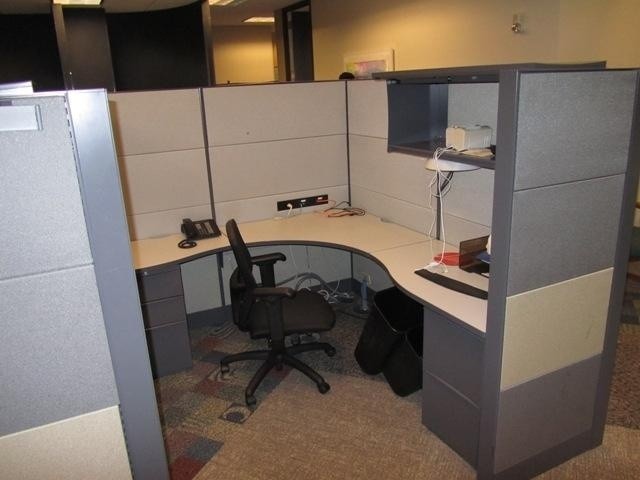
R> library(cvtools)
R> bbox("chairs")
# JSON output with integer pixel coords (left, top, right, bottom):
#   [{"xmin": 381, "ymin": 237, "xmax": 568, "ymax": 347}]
[{"xmin": 221, "ymin": 218, "xmax": 338, "ymax": 411}]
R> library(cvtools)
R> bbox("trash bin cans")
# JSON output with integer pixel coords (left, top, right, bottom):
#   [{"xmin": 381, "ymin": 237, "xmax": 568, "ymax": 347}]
[{"xmin": 354, "ymin": 287, "xmax": 423, "ymax": 398}]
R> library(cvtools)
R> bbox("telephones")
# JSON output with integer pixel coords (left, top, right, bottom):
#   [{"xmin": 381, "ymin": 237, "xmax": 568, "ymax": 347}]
[{"xmin": 181, "ymin": 218, "xmax": 221, "ymax": 241}]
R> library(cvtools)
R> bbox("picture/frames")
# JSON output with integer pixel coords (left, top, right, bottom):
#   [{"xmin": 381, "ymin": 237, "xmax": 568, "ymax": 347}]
[{"xmin": 342, "ymin": 48, "xmax": 394, "ymax": 76}]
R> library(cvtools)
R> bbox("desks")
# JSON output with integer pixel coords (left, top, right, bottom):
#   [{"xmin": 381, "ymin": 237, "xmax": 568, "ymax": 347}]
[{"xmin": 131, "ymin": 209, "xmax": 489, "ymax": 471}]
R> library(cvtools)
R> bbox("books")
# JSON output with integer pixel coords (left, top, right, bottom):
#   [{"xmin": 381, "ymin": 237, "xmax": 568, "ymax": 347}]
[{"xmin": 0, "ymin": 79, "xmax": 35, "ymax": 96}]
